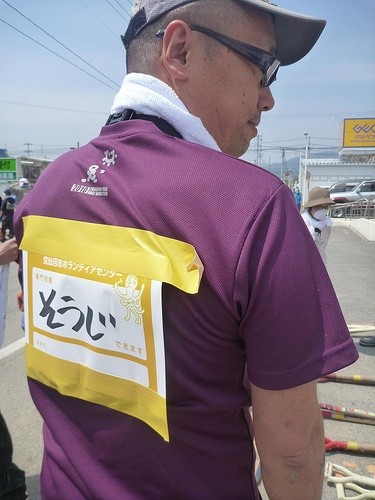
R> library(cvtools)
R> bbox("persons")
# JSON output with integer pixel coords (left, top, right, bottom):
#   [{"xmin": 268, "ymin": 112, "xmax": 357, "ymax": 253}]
[
  {"xmin": 0, "ymin": 188, "xmax": 15, "ymax": 242},
  {"xmin": 297, "ymin": 186, "xmax": 335, "ymax": 261},
  {"xmin": 12, "ymin": 0, "xmax": 360, "ymax": 499},
  {"xmin": 10, "ymin": 177, "xmax": 33, "ymax": 214},
  {"xmin": 291, "ymin": 188, "xmax": 303, "ymax": 213},
  {"xmin": 0, "ymin": 237, "xmax": 19, "ymax": 265}
]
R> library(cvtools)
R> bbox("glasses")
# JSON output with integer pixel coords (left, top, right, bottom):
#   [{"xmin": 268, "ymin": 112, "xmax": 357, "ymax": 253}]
[{"xmin": 155, "ymin": 24, "xmax": 282, "ymax": 89}]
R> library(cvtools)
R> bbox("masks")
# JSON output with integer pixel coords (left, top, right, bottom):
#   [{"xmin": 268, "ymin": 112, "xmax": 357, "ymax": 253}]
[{"xmin": 312, "ymin": 209, "xmax": 328, "ymax": 221}]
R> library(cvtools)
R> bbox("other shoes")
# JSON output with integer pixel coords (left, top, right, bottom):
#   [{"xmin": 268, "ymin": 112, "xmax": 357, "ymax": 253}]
[{"xmin": 0, "ymin": 478, "xmax": 29, "ymax": 500}]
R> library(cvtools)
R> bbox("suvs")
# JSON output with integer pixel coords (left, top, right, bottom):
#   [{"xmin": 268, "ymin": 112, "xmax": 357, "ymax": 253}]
[{"xmin": 328, "ymin": 180, "xmax": 375, "ymax": 217}]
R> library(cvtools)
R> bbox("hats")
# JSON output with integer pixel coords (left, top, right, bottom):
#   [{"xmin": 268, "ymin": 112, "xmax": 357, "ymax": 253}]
[
  {"xmin": 120, "ymin": 1, "xmax": 327, "ymax": 66},
  {"xmin": 304, "ymin": 186, "xmax": 335, "ymax": 208}
]
